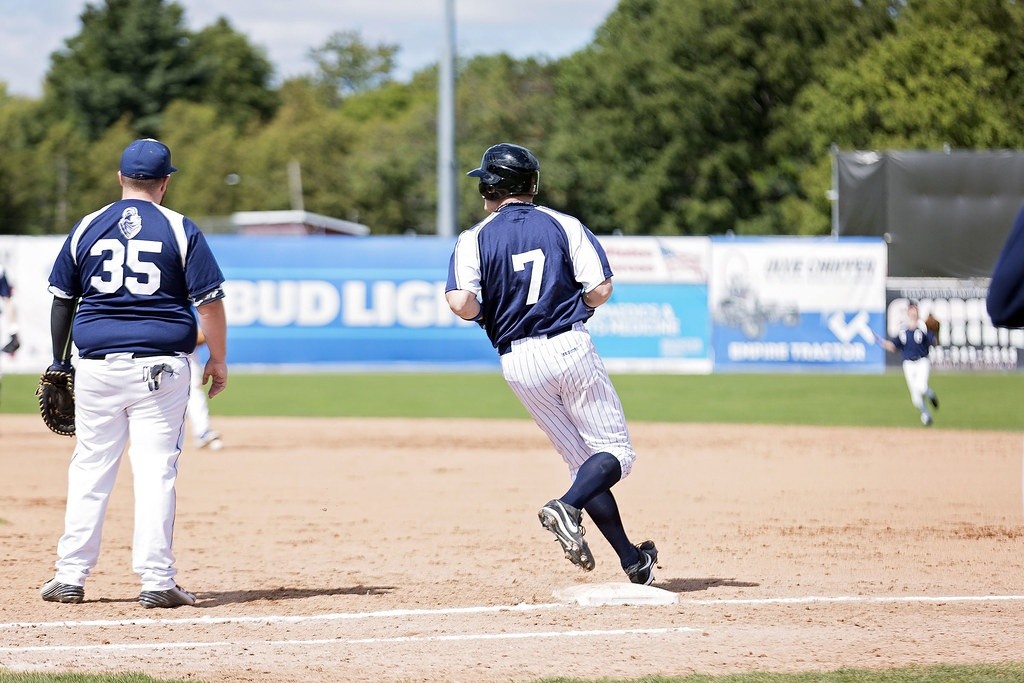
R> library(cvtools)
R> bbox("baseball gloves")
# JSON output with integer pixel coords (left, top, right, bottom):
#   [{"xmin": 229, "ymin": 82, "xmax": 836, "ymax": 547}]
[{"xmin": 35, "ymin": 355, "xmax": 75, "ymax": 437}]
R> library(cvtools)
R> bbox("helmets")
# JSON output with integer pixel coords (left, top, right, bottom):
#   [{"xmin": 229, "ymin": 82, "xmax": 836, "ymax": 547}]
[{"xmin": 466, "ymin": 144, "xmax": 540, "ymax": 201}]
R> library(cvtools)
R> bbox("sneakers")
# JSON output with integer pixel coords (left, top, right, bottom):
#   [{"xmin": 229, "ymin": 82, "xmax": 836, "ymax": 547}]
[
  {"xmin": 919, "ymin": 396, "xmax": 937, "ymax": 427},
  {"xmin": 623, "ymin": 541, "xmax": 658, "ymax": 586},
  {"xmin": 137, "ymin": 586, "xmax": 198, "ymax": 609},
  {"xmin": 538, "ymin": 500, "xmax": 595, "ymax": 572},
  {"xmin": 41, "ymin": 579, "xmax": 84, "ymax": 603}
]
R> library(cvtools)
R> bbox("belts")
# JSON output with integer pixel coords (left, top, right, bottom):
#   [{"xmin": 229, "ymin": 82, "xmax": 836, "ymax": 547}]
[
  {"xmin": 84, "ymin": 352, "xmax": 180, "ymax": 361},
  {"xmin": 497, "ymin": 326, "xmax": 572, "ymax": 356}
]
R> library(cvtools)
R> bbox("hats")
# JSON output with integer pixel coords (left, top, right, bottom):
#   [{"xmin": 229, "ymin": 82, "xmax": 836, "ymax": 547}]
[{"xmin": 119, "ymin": 138, "xmax": 177, "ymax": 180}]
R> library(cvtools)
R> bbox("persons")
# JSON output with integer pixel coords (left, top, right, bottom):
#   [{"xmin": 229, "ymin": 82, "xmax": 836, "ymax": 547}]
[
  {"xmin": 877, "ymin": 301, "xmax": 941, "ymax": 425},
  {"xmin": 186, "ymin": 322, "xmax": 225, "ymax": 452},
  {"xmin": 43, "ymin": 138, "xmax": 228, "ymax": 608},
  {"xmin": 444, "ymin": 145, "xmax": 659, "ymax": 585}
]
